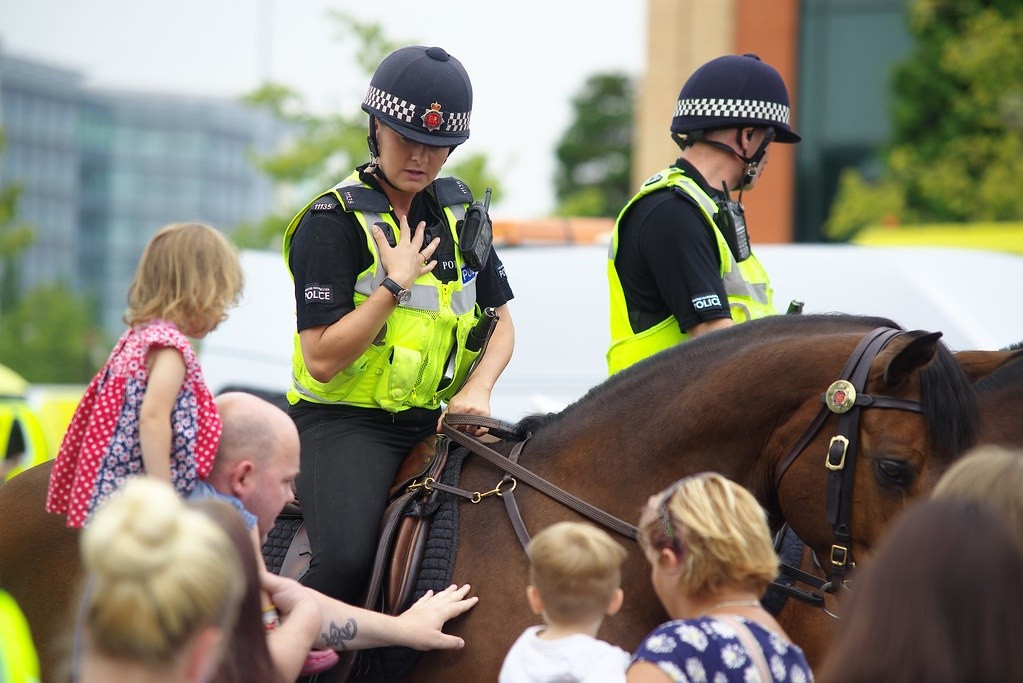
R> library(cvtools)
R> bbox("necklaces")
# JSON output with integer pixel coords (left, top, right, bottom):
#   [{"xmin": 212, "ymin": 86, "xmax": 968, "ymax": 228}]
[{"xmin": 690, "ymin": 598, "xmax": 763, "ymax": 622}]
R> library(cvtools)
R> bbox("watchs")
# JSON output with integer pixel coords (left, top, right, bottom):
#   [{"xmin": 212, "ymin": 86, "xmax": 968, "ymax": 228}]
[{"xmin": 380, "ymin": 277, "xmax": 412, "ymax": 306}]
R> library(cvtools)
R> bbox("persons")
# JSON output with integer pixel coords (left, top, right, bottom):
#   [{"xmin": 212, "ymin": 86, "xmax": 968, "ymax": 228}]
[
  {"xmin": 498, "ymin": 520, "xmax": 632, "ymax": 683},
  {"xmin": 606, "ymin": 52, "xmax": 803, "ymax": 385},
  {"xmin": 280, "ymin": 45, "xmax": 514, "ymax": 610},
  {"xmin": 204, "ymin": 391, "xmax": 479, "ymax": 682},
  {"xmin": 69, "ymin": 479, "xmax": 244, "ymax": 683},
  {"xmin": 46, "ymin": 224, "xmax": 341, "ymax": 674},
  {"xmin": 623, "ymin": 470, "xmax": 814, "ymax": 683}
]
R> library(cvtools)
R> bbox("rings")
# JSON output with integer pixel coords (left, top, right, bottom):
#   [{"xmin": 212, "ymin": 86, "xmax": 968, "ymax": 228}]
[{"xmin": 420, "ymin": 251, "xmax": 428, "ymax": 264}]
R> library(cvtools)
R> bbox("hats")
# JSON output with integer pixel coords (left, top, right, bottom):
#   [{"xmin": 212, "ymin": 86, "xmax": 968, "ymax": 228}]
[
  {"xmin": 361, "ymin": 47, "xmax": 472, "ymax": 146},
  {"xmin": 670, "ymin": 51, "xmax": 803, "ymax": 144}
]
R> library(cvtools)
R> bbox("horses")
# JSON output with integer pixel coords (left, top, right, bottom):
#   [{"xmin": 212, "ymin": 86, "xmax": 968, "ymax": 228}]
[{"xmin": 3, "ymin": 313, "xmax": 977, "ymax": 683}]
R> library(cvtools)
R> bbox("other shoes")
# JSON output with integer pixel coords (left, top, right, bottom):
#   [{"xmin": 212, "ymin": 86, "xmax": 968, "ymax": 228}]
[{"xmin": 298, "ymin": 648, "xmax": 339, "ymax": 677}]
